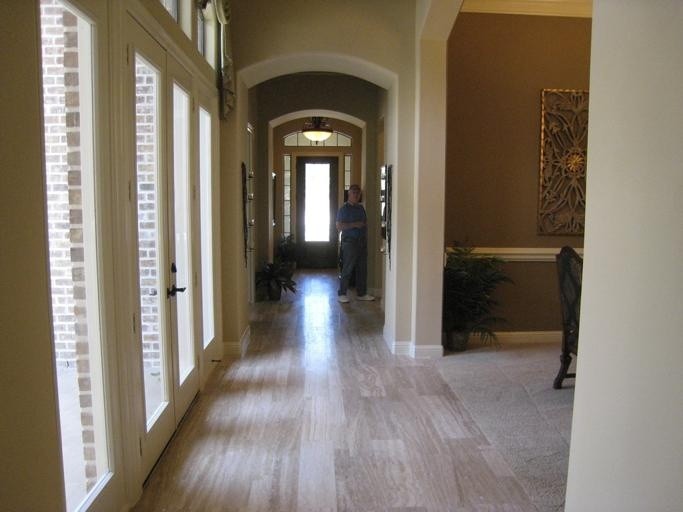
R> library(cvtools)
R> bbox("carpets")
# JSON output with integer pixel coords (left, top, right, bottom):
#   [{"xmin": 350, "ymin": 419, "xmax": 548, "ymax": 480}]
[{"xmin": 436, "ymin": 343, "xmax": 577, "ymax": 512}]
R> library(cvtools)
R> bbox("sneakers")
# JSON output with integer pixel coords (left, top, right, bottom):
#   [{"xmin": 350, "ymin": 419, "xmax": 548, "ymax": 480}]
[
  {"xmin": 338, "ymin": 294, "xmax": 350, "ymax": 303},
  {"xmin": 355, "ymin": 294, "xmax": 375, "ymax": 301}
]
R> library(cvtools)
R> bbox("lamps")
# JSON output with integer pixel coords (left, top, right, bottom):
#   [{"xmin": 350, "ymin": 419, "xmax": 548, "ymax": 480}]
[
  {"xmin": 302, "ymin": 117, "xmax": 333, "ymax": 145},
  {"xmin": 242, "ymin": 161, "xmax": 255, "ymax": 251}
]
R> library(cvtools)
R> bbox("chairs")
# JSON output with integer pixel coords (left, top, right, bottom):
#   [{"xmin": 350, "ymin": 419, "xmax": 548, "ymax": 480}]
[{"xmin": 554, "ymin": 245, "xmax": 583, "ymax": 390}]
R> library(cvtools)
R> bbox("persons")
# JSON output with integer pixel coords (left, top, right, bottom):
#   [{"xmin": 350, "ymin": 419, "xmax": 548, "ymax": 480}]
[{"xmin": 334, "ymin": 184, "xmax": 375, "ymax": 304}]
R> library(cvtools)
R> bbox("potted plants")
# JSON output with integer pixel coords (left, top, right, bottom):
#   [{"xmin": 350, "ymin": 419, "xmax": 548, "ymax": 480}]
[
  {"xmin": 256, "ymin": 261, "xmax": 296, "ymax": 301},
  {"xmin": 442, "ymin": 242, "xmax": 515, "ymax": 351},
  {"xmin": 277, "ymin": 235, "xmax": 313, "ymax": 270}
]
